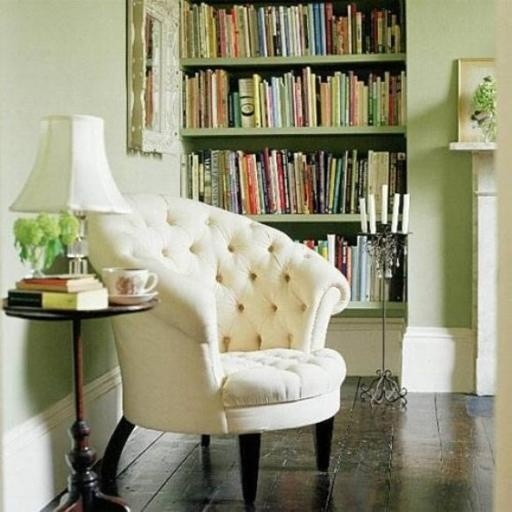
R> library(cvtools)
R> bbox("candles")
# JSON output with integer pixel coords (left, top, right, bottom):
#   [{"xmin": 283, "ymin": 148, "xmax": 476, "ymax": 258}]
[
  {"xmin": 381, "ymin": 185, "xmax": 388, "ymax": 224},
  {"xmin": 360, "ymin": 199, "xmax": 367, "ymax": 233},
  {"xmin": 368, "ymin": 195, "xmax": 376, "ymax": 233},
  {"xmin": 403, "ymin": 194, "xmax": 410, "ymax": 233},
  {"xmin": 391, "ymin": 194, "xmax": 400, "ymax": 232}
]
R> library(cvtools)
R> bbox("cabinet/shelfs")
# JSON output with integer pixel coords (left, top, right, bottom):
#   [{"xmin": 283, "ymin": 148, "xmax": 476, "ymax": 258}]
[{"xmin": 185, "ymin": 0, "xmax": 406, "ymax": 311}]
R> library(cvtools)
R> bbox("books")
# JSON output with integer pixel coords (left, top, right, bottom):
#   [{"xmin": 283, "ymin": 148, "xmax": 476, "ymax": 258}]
[
  {"xmin": 181, "ymin": 145, "xmax": 406, "ymax": 214},
  {"xmin": 183, "ymin": 0, "xmax": 401, "ymax": 59},
  {"xmin": 182, "ymin": 66, "xmax": 406, "ymax": 129},
  {"xmin": 7, "ymin": 273, "xmax": 109, "ymax": 311},
  {"xmin": 295, "ymin": 233, "xmax": 407, "ymax": 302}
]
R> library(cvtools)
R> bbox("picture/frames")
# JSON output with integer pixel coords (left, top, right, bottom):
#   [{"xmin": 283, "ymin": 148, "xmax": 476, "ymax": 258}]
[{"xmin": 457, "ymin": 58, "xmax": 496, "ymax": 143}]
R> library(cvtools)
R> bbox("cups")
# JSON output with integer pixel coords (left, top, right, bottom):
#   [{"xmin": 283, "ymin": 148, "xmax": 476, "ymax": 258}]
[{"xmin": 102, "ymin": 266, "xmax": 159, "ymax": 295}]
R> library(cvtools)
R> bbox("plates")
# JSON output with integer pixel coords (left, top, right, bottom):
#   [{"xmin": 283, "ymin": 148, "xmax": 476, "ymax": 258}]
[{"xmin": 109, "ymin": 291, "xmax": 159, "ymax": 304}]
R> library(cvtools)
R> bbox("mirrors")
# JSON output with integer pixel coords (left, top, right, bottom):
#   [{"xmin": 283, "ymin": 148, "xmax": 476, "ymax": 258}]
[{"xmin": 127, "ymin": 0, "xmax": 182, "ymax": 156}]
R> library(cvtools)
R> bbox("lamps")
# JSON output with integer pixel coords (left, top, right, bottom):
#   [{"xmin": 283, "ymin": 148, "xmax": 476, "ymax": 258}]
[{"xmin": 10, "ymin": 115, "xmax": 130, "ymax": 278}]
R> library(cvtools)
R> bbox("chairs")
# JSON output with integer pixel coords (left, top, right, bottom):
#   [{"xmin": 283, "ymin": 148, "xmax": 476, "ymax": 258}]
[{"xmin": 86, "ymin": 195, "xmax": 351, "ymax": 503}]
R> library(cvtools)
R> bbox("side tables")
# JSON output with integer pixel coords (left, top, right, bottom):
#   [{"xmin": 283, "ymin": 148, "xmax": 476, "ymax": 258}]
[{"xmin": 1, "ymin": 301, "xmax": 160, "ymax": 512}]
